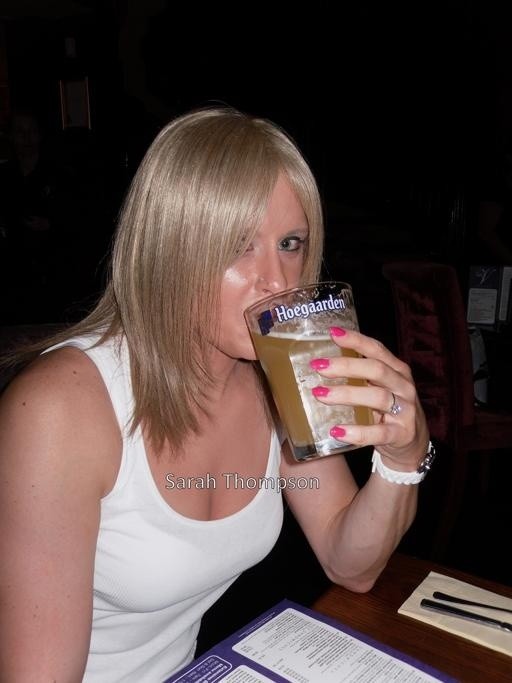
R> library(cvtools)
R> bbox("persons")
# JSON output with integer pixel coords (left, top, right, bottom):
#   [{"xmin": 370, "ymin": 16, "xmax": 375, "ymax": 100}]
[{"xmin": 2, "ymin": 107, "xmax": 437, "ymax": 681}]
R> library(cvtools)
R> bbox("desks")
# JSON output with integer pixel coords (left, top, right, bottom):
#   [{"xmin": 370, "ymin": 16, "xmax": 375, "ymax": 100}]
[{"xmin": 313, "ymin": 552, "xmax": 512, "ymax": 683}]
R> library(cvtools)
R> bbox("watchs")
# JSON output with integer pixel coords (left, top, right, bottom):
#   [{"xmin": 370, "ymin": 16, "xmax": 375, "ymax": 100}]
[{"xmin": 370, "ymin": 440, "xmax": 437, "ymax": 487}]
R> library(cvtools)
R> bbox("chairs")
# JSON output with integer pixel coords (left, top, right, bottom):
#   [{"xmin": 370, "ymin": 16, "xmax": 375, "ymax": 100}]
[{"xmin": 383, "ymin": 262, "xmax": 512, "ymax": 546}]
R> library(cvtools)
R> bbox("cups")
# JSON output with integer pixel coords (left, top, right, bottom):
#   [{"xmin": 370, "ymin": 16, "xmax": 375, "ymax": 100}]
[{"xmin": 243, "ymin": 278, "xmax": 376, "ymax": 467}]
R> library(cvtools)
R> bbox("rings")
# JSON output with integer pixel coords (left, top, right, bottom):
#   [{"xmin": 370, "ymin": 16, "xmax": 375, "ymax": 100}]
[{"xmin": 389, "ymin": 392, "xmax": 402, "ymax": 417}]
